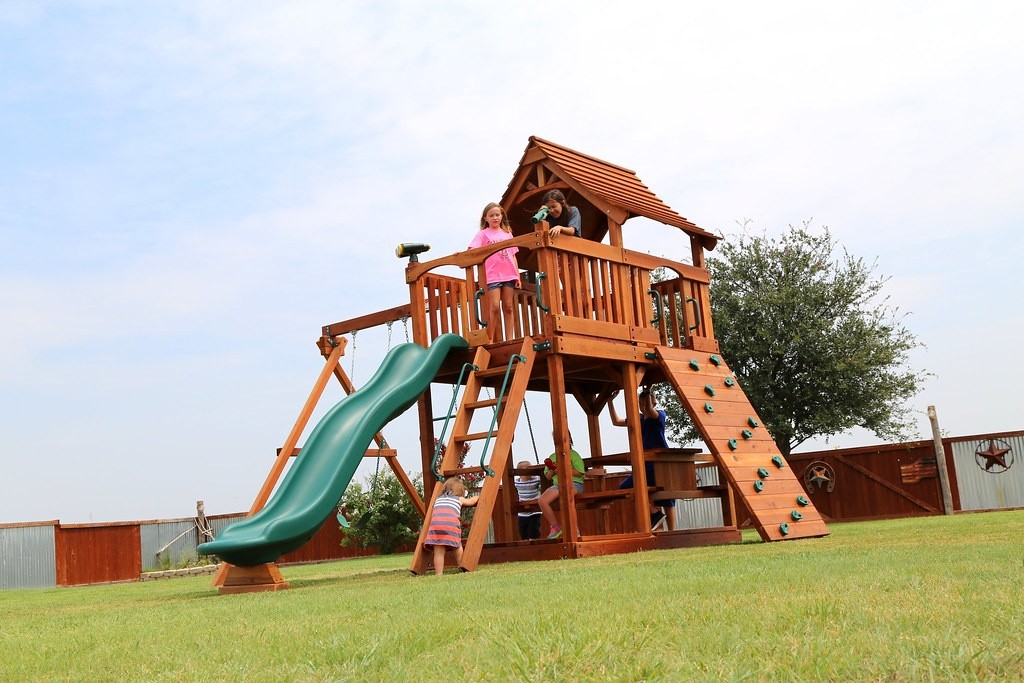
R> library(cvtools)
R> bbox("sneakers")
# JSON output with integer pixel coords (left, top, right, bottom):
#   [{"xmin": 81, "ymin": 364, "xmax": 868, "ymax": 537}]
[
  {"xmin": 577, "ymin": 526, "xmax": 581, "ymax": 537},
  {"xmin": 650, "ymin": 510, "xmax": 667, "ymax": 531},
  {"xmin": 547, "ymin": 524, "xmax": 562, "ymax": 539}
]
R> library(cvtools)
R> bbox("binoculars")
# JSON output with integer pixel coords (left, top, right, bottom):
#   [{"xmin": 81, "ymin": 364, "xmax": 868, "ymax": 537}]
[{"xmin": 395, "ymin": 242, "xmax": 431, "ymax": 258}]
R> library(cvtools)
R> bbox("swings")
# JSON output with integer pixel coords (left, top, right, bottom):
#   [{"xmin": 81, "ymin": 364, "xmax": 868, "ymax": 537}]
[{"xmin": 335, "ymin": 327, "xmax": 393, "ymax": 530}]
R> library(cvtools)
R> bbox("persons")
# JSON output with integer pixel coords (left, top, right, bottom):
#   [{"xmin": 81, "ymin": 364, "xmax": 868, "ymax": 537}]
[
  {"xmin": 537, "ymin": 429, "xmax": 585, "ymax": 540},
  {"xmin": 539, "ymin": 188, "xmax": 587, "ymax": 320},
  {"xmin": 608, "ymin": 382, "xmax": 675, "ymax": 530},
  {"xmin": 514, "ymin": 461, "xmax": 544, "ymax": 539},
  {"xmin": 454, "ymin": 203, "xmax": 522, "ymax": 345},
  {"xmin": 423, "ymin": 477, "xmax": 479, "ymax": 574}
]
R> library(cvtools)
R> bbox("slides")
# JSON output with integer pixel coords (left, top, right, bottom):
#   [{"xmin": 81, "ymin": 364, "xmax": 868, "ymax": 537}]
[{"xmin": 192, "ymin": 331, "xmax": 470, "ymax": 568}]
